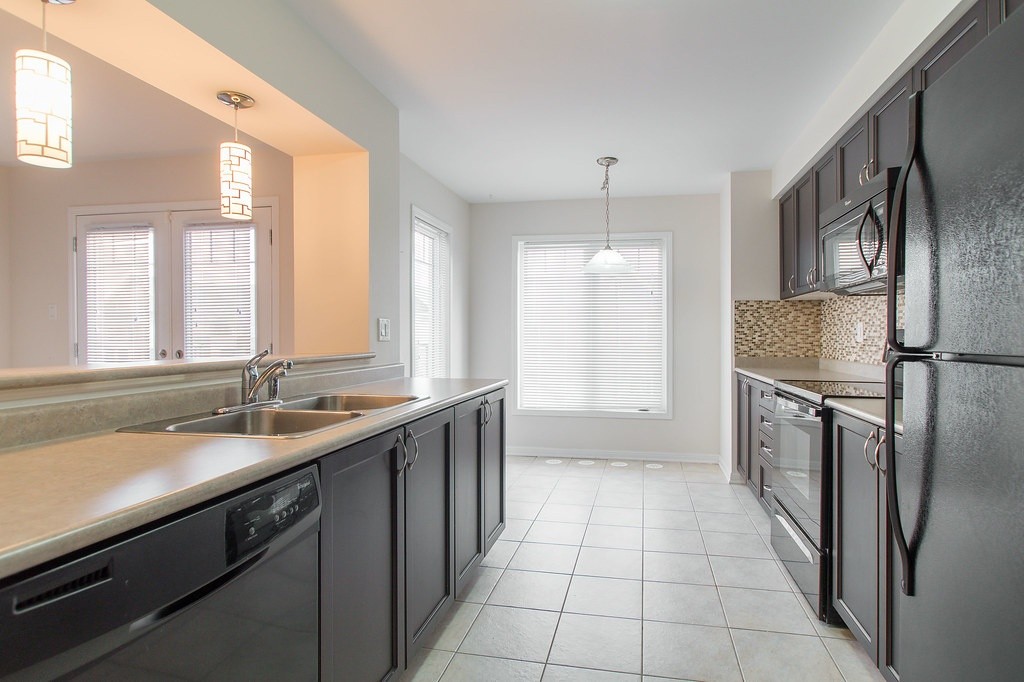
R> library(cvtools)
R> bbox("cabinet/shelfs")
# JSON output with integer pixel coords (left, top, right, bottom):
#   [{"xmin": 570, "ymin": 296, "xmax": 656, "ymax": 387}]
[
  {"xmin": 833, "ymin": 410, "xmax": 910, "ymax": 682},
  {"xmin": 779, "ymin": 187, "xmax": 797, "ymax": 299},
  {"xmin": 319, "ymin": 403, "xmax": 454, "ymax": 682},
  {"xmin": 912, "ymin": 0, "xmax": 1024, "ymax": 92},
  {"xmin": 736, "ymin": 371, "xmax": 759, "ymax": 502},
  {"xmin": 454, "ymin": 381, "xmax": 509, "ymax": 611},
  {"xmin": 836, "ymin": 68, "xmax": 913, "ymax": 200},
  {"xmin": 759, "ymin": 381, "xmax": 782, "ymax": 520},
  {"xmin": 793, "ymin": 144, "xmax": 836, "ymax": 296}
]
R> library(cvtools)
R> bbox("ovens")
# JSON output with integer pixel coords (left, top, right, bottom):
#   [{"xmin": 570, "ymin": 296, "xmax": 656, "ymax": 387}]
[{"xmin": 769, "ymin": 389, "xmax": 831, "ymax": 623}]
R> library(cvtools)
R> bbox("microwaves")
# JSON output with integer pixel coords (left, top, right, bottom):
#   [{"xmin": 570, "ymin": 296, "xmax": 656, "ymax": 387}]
[{"xmin": 820, "ymin": 167, "xmax": 905, "ymax": 299}]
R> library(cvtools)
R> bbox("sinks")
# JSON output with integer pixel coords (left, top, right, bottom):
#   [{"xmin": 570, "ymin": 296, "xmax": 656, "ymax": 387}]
[
  {"xmin": 257, "ymin": 391, "xmax": 430, "ymax": 414},
  {"xmin": 116, "ymin": 401, "xmax": 371, "ymax": 439}
]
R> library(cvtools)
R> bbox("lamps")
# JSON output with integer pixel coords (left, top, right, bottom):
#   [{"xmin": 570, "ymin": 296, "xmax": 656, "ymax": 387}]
[
  {"xmin": 216, "ymin": 91, "xmax": 254, "ymax": 220},
  {"xmin": 15, "ymin": 0, "xmax": 73, "ymax": 168},
  {"xmin": 581, "ymin": 156, "xmax": 634, "ymax": 273}
]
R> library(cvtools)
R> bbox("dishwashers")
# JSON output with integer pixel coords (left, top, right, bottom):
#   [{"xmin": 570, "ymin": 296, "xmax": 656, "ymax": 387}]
[{"xmin": 1, "ymin": 465, "xmax": 324, "ymax": 680}]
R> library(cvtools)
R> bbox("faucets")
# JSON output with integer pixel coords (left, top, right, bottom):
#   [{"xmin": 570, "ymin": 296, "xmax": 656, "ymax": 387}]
[
  {"xmin": 266, "ymin": 369, "xmax": 288, "ymax": 401},
  {"xmin": 241, "ymin": 349, "xmax": 293, "ymax": 405}
]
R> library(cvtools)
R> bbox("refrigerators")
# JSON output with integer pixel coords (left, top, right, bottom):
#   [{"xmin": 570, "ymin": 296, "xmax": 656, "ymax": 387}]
[{"xmin": 885, "ymin": 3, "xmax": 1023, "ymax": 682}]
